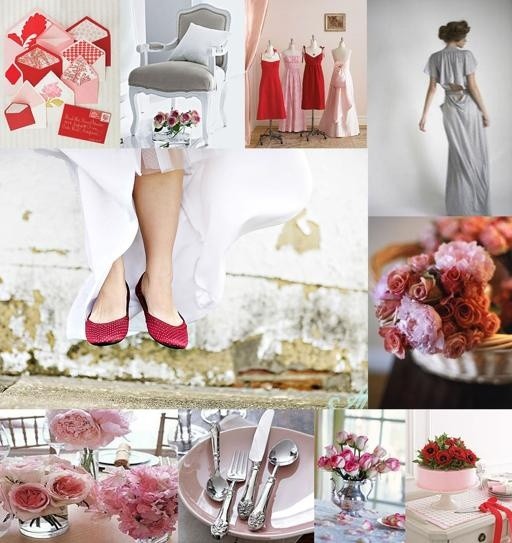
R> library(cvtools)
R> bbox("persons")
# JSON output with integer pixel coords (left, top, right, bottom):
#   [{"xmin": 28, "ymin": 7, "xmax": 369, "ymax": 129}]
[
  {"xmin": 416, "ymin": 17, "xmax": 494, "ymax": 218},
  {"xmin": 256, "ymin": 41, "xmax": 285, "ymax": 119},
  {"xmin": 300, "ymin": 38, "xmax": 325, "ymax": 111},
  {"xmin": 329, "ymin": 40, "xmax": 353, "ymax": 136},
  {"xmin": 85, "ymin": 150, "xmax": 188, "ymax": 351},
  {"xmin": 277, "ymin": 40, "xmax": 307, "ymax": 132}
]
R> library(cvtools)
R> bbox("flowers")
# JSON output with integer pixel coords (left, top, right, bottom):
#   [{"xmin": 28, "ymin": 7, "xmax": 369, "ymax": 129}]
[
  {"xmin": 152, "ymin": 104, "xmax": 200, "ymax": 147},
  {"xmin": 411, "ymin": 431, "xmax": 481, "ymax": 471},
  {"xmin": 0, "ymin": 453, "xmax": 98, "ymax": 531},
  {"xmin": 372, "ymin": 215, "xmax": 510, "ymax": 361},
  {"xmin": 45, "ymin": 408, "xmax": 132, "ymax": 480},
  {"xmin": 93, "ymin": 464, "xmax": 179, "ymax": 540},
  {"xmin": 316, "ymin": 429, "xmax": 400, "ymax": 481}
]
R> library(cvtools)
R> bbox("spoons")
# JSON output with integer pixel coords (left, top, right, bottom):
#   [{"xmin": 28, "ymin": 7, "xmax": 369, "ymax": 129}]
[
  {"xmin": 207, "ymin": 422, "xmax": 230, "ymax": 502},
  {"xmin": 247, "ymin": 438, "xmax": 299, "ymax": 532}
]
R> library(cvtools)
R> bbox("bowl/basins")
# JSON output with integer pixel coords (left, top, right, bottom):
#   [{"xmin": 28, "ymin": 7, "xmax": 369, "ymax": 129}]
[{"xmin": 412, "ymin": 340, "xmax": 512, "ymax": 385}]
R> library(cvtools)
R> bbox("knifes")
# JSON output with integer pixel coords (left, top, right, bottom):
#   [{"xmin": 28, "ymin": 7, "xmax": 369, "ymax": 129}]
[{"xmin": 237, "ymin": 409, "xmax": 276, "ymax": 521}]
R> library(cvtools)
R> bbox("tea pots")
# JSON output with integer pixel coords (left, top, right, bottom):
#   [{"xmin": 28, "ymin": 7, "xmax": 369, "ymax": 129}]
[{"xmin": 328, "ymin": 477, "xmax": 373, "ymax": 518}]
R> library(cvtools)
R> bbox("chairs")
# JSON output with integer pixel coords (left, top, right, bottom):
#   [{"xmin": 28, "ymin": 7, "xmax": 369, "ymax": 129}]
[{"xmin": 125, "ymin": 1, "xmax": 232, "ymax": 147}]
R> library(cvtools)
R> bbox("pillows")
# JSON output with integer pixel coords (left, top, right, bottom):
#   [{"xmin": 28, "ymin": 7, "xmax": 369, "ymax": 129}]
[{"xmin": 167, "ymin": 21, "xmax": 234, "ymax": 66}]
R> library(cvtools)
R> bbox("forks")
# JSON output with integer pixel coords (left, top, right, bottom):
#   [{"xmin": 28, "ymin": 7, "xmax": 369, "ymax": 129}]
[{"xmin": 209, "ymin": 449, "xmax": 249, "ymax": 539}]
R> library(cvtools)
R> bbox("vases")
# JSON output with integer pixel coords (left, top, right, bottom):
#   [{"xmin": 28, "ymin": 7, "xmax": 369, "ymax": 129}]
[
  {"xmin": 19, "ymin": 504, "xmax": 70, "ymax": 538},
  {"xmin": 76, "ymin": 447, "xmax": 100, "ymax": 481}
]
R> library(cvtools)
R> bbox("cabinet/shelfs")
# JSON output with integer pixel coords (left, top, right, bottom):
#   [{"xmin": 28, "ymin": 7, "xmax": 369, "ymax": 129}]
[{"xmin": 405, "ymin": 512, "xmax": 511, "ymax": 542}]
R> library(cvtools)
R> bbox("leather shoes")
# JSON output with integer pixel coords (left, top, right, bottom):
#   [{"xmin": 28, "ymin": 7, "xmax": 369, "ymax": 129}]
[
  {"xmin": 85, "ymin": 280, "xmax": 130, "ymax": 346},
  {"xmin": 135, "ymin": 271, "xmax": 188, "ymax": 349}
]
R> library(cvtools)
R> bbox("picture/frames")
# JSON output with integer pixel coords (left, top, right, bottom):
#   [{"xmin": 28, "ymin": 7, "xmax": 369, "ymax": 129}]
[{"xmin": 324, "ymin": 12, "xmax": 347, "ymax": 32}]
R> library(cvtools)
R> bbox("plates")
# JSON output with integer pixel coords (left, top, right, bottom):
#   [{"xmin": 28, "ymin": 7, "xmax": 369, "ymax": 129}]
[{"xmin": 178, "ymin": 423, "xmax": 314, "ymax": 540}]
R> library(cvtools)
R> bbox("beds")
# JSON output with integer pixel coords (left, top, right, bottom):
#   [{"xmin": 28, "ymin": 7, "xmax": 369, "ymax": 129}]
[{"xmin": 1, "ymin": 452, "xmax": 178, "ymax": 542}]
